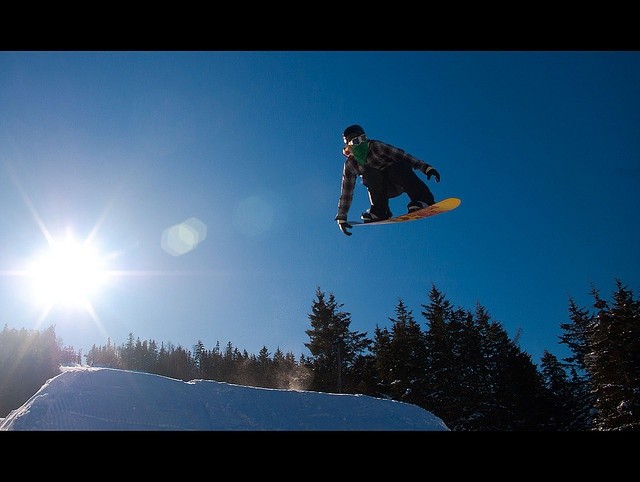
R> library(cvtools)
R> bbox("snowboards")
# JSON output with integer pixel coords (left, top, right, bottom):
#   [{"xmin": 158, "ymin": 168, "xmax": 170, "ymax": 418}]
[{"xmin": 348, "ymin": 197, "xmax": 461, "ymax": 225}]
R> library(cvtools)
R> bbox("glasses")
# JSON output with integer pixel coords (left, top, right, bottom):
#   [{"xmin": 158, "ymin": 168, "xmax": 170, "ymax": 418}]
[{"xmin": 352, "ymin": 134, "xmax": 367, "ymax": 144}]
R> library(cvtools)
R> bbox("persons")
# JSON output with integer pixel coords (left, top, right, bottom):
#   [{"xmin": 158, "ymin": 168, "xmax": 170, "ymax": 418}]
[{"xmin": 336, "ymin": 125, "xmax": 439, "ymax": 236}]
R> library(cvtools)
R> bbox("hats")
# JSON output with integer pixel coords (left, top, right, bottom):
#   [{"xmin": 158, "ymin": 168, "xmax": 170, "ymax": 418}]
[{"xmin": 342, "ymin": 125, "xmax": 365, "ymax": 143}]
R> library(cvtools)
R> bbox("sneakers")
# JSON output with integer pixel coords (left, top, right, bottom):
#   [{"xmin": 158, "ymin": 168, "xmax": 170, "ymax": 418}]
[
  {"xmin": 407, "ymin": 200, "xmax": 437, "ymax": 212},
  {"xmin": 361, "ymin": 206, "xmax": 393, "ymax": 221}
]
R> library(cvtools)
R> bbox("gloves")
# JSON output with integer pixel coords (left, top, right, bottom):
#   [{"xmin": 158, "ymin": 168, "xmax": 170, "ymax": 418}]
[
  {"xmin": 423, "ymin": 164, "xmax": 441, "ymax": 183},
  {"xmin": 337, "ymin": 220, "xmax": 353, "ymax": 236}
]
